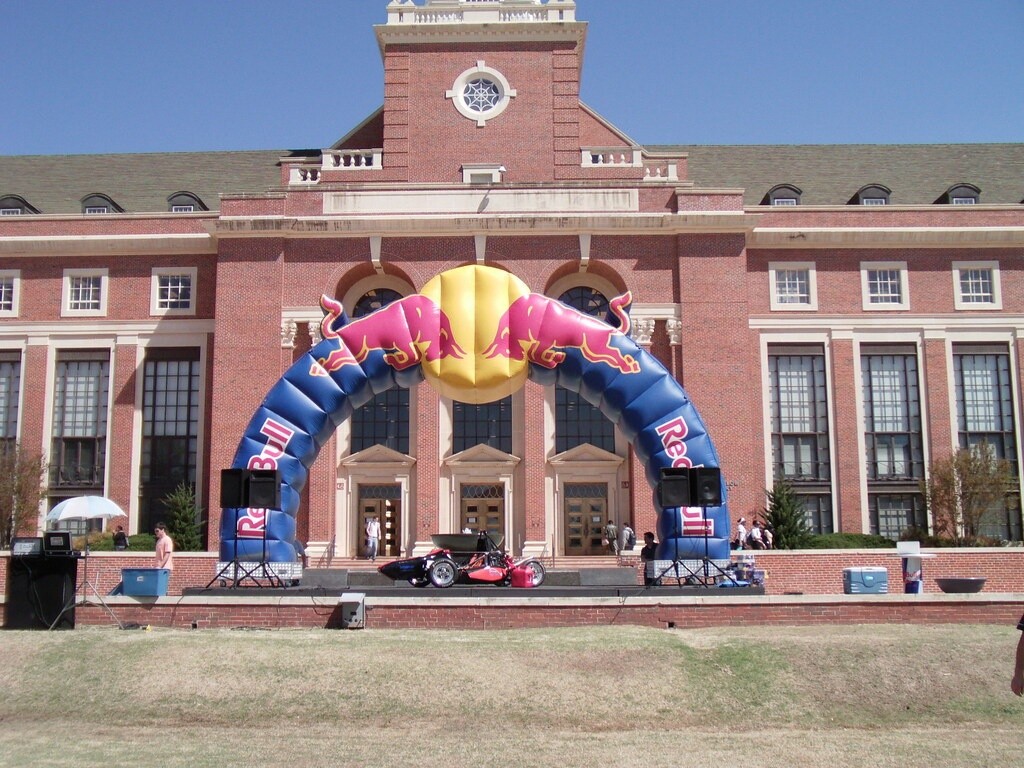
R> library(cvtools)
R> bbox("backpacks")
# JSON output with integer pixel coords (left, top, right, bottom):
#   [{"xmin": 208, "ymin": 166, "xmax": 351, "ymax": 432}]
[{"xmin": 624, "ymin": 529, "xmax": 636, "ymax": 545}]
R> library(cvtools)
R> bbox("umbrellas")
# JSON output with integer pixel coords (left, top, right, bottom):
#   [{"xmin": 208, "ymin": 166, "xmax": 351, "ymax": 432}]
[{"xmin": 45, "ymin": 492, "xmax": 129, "ymax": 605}]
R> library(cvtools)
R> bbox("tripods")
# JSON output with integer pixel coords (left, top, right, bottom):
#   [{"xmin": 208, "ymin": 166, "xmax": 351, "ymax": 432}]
[
  {"xmin": 205, "ymin": 509, "xmax": 286, "ymax": 589},
  {"xmin": 649, "ymin": 508, "xmax": 739, "ymax": 589}
]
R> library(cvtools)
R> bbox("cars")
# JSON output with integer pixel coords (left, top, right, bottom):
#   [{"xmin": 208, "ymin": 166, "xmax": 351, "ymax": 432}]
[{"xmin": 378, "ymin": 528, "xmax": 546, "ymax": 589}]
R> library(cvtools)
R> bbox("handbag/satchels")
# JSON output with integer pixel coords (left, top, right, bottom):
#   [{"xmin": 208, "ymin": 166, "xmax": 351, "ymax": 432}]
[
  {"xmin": 747, "ymin": 534, "xmax": 753, "ymax": 544},
  {"xmin": 602, "ymin": 539, "xmax": 609, "ymax": 545}
]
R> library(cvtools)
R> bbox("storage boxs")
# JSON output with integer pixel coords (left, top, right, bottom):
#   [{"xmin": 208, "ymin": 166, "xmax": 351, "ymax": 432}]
[
  {"xmin": 843, "ymin": 567, "xmax": 888, "ymax": 594},
  {"xmin": 122, "ymin": 568, "xmax": 170, "ymax": 595}
]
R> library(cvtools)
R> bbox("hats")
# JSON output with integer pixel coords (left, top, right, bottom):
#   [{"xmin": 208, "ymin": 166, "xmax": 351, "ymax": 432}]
[{"xmin": 738, "ymin": 518, "xmax": 744, "ymax": 523}]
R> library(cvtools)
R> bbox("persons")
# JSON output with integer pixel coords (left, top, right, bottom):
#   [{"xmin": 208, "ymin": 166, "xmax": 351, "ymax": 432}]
[
  {"xmin": 366, "ymin": 515, "xmax": 382, "ymax": 561},
  {"xmin": 750, "ymin": 520, "xmax": 767, "ymax": 550},
  {"xmin": 734, "ymin": 517, "xmax": 747, "ymax": 549},
  {"xmin": 621, "ymin": 521, "xmax": 634, "ymax": 551},
  {"xmin": 112, "ymin": 525, "xmax": 129, "ymax": 551},
  {"xmin": 604, "ymin": 520, "xmax": 619, "ymax": 556},
  {"xmin": 641, "ymin": 531, "xmax": 662, "ymax": 585},
  {"xmin": 153, "ymin": 521, "xmax": 173, "ymax": 571},
  {"xmin": 1011, "ymin": 613, "xmax": 1024, "ymax": 698},
  {"xmin": 763, "ymin": 521, "xmax": 776, "ymax": 550}
]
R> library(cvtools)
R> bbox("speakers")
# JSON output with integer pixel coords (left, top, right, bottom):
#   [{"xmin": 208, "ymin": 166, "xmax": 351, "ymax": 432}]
[
  {"xmin": 690, "ymin": 467, "xmax": 721, "ymax": 507},
  {"xmin": 219, "ymin": 468, "xmax": 249, "ymax": 509},
  {"xmin": 248, "ymin": 469, "xmax": 281, "ymax": 509},
  {"xmin": 658, "ymin": 467, "xmax": 690, "ymax": 508}
]
